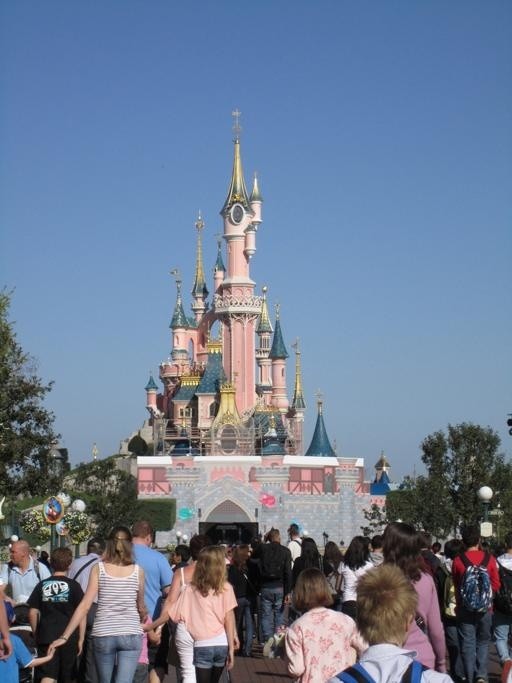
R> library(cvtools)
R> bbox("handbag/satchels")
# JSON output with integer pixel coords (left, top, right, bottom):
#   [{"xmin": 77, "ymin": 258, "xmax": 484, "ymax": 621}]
[
  {"xmin": 167, "ymin": 566, "xmax": 187, "ymax": 625},
  {"xmin": 326, "ymin": 572, "xmax": 338, "ymax": 595},
  {"xmin": 166, "ymin": 633, "xmax": 177, "ymax": 666},
  {"xmin": 243, "ymin": 572, "xmax": 258, "ymax": 606},
  {"xmin": 222, "ymin": 666, "xmax": 232, "ymax": 683}
]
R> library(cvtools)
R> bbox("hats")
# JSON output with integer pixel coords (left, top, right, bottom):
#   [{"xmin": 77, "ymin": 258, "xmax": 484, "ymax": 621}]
[{"xmin": 288, "ymin": 524, "xmax": 299, "ymax": 532}]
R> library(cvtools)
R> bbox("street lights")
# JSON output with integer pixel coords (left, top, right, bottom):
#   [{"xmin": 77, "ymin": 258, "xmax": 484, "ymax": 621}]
[
  {"xmin": 182, "ymin": 534, "xmax": 189, "ymax": 547},
  {"xmin": 54, "ymin": 489, "xmax": 70, "ymax": 548},
  {"xmin": 175, "ymin": 531, "xmax": 181, "ymax": 545},
  {"xmin": 71, "ymin": 498, "xmax": 86, "ymax": 559},
  {"xmin": 479, "ymin": 484, "xmax": 496, "ymax": 547},
  {"xmin": 35, "ymin": 544, "xmax": 41, "ymax": 558},
  {"xmin": 46, "ymin": 439, "xmax": 63, "ymax": 552},
  {"xmin": 303, "ymin": 529, "xmax": 308, "ymax": 537}
]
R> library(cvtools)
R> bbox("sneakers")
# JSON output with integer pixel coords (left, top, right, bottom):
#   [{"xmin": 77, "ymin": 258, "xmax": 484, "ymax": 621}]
[
  {"xmin": 456, "ymin": 676, "xmax": 489, "ymax": 683},
  {"xmin": 500, "ymin": 659, "xmax": 512, "ymax": 683},
  {"xmin": 235, "ymin": 633, "xmax": 284, "ymax": 660}
]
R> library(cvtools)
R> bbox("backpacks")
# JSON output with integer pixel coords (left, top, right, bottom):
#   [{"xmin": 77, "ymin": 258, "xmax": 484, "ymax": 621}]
[
  {"xmin": 458, "ymin": 550, "xmax": 494, "ymax": 614},
  {"xmin": 494, "ymin": 559, "xmax": 512, "ymax": 615},
  {"xmin": 439, "ymin": 562, "xmax": 457, "ymax": 617}
]
